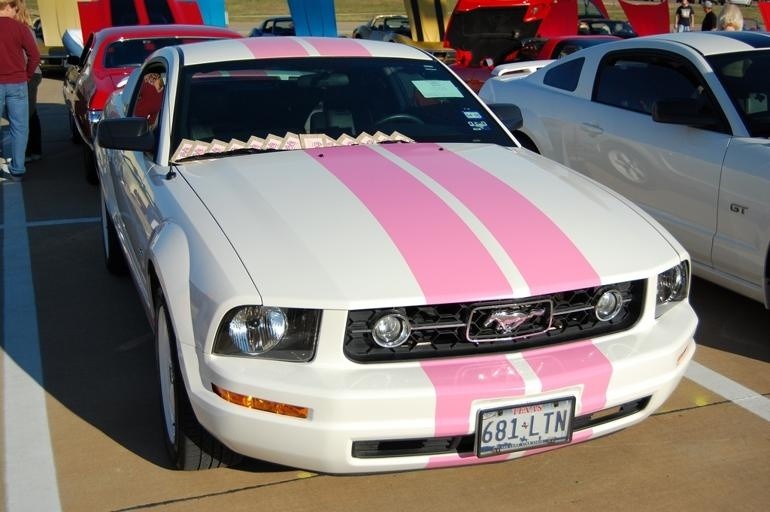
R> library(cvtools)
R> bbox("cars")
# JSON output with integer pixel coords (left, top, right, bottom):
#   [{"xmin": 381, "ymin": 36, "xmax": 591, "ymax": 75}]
[
  {"xmin": 352, "ymin": 14, "xmax": 413, "ymax": 43},
  {"xmin": 437, "ymin": 0, "xmax": 625, "ymax": 95},
  {"xmin": 578, "ymin": 18, "xmax": 637, "ymax": 39},
  {"xmin": 478, "ymin": 30, "xmax": 770, "ymax": 313},
  {"xmin": 98, "ymin": 35, "xmax": 699, "ymax": 477},
  {"xmin": 249, "ymin": 16, "xmax": 297, "ymax": 36},
  {"xmin": 62, "ymin": 23, "xmax": 269, "ymax": 182}
]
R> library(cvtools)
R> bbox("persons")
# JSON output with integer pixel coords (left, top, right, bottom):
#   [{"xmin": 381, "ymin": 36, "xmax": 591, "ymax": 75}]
[
  {"xmin": 0, "ymin": 0, "xmax": 43, "ymax": 182},
  {"xmin": 134, "ymin": 72, "xmax": 166, "ymax": 129},
  {"xmin": 674, "ymin": 0, "xmax": 744, "ymax": 33}
]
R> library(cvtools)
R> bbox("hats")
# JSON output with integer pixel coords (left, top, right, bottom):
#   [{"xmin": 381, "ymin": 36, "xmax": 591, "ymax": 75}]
[{"xmin": 702, "ymin": 1, "xmax": 712, "ymax": 9}]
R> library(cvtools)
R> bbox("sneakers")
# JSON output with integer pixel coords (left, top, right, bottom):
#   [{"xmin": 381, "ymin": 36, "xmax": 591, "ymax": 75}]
[{"xmin": 0, "ymin": 152, "xmax": 42, "ymax": 182}]
